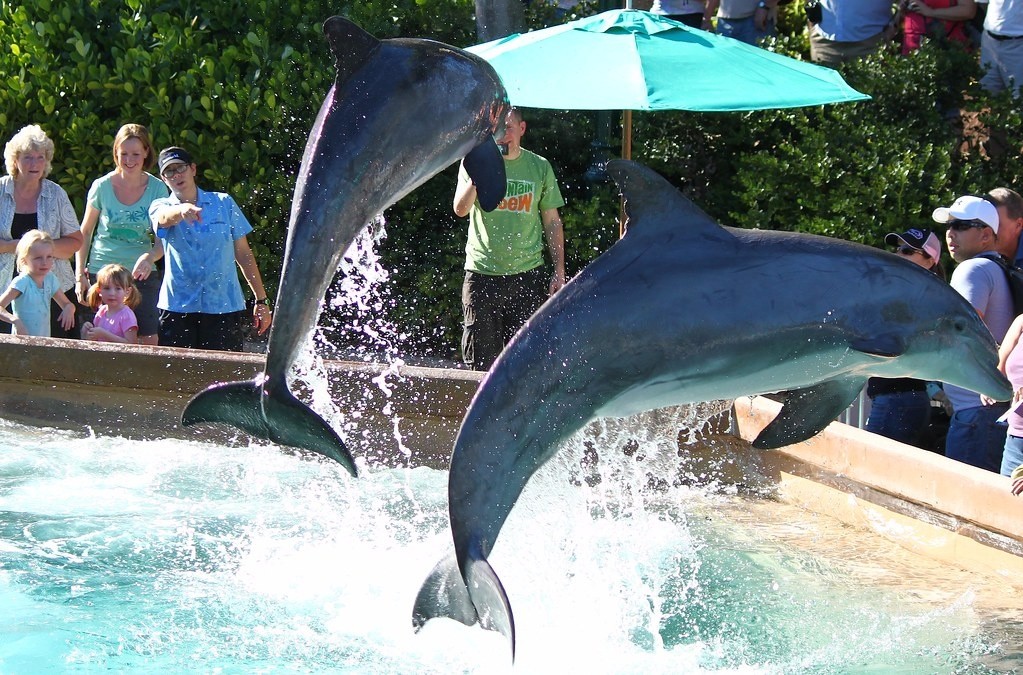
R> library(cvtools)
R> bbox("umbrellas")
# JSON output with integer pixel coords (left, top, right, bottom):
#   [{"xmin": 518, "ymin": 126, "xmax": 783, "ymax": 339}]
[{"xmin": 457, "ymin": 0, "xmax": 872, "ymax": 239}]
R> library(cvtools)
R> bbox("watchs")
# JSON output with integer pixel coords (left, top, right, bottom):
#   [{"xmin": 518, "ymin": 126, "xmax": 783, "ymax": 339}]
[
  {"xmin": 254, "ymin": 298, "xmax": 270, "ymax": 305},
  {"xmin": 758, "ymin": 0, "xmax": 769, "ymax": 11}
]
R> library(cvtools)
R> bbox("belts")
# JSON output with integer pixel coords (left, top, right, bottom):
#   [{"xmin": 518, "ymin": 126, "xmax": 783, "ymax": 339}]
[{"xmin": 986, "ymin": 30, "xmax": 1023, "ymax": 40}]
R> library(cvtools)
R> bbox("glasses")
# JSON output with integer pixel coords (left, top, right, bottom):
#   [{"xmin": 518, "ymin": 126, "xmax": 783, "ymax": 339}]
[
  {"xmin": 944, "ymin": 220, "xmax": 986, "ymax": 231},
  {"xmin": 161, "ymin": 163, "xmax": 192, "ymax": 178},
  {"xmin": 894, "ymin": 243, "xmax": 928, "ymax": 257}
]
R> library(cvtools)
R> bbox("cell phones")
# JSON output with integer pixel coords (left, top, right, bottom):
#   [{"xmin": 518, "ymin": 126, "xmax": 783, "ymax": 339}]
[{"xmin": 498, "ymin": 144, "xmax": 509, "ymax": 155}]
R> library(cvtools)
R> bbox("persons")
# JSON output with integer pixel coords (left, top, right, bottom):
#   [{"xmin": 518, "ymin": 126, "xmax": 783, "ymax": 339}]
[
  {"xmin": 74, "ymin": 124, "xmax": 164, "ymax": 346},
  {"xmin": 452, "ymin": 102, "xmax": 565, "ymax": 372},
  {"xmin": 149, "ymin": 147, "xmax": 271, "ymax": 352},
  {"xmin": 0, "ymin": 125, "xmax": 83, "ymax": 340},
  {"xmin": 0, "ymin": 229, "xmax": 76, "ymax": 338},
  {"xmin": 648, "ymin": 0, "xmax": 1023, "ymax": 496},
  {"xmin": 82, "ymin": 263, "xmax": 142, "ymax": 343}
]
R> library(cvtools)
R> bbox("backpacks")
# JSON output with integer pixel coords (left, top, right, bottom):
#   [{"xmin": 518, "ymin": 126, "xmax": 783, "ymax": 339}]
[{"xmin": 986, "ymin": 252, "xmax": 1023, "ymax": 314}]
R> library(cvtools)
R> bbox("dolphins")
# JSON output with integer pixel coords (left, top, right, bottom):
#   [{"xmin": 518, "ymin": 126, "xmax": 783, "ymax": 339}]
[
  {"xmin": 409, "ymin": 156, "xmax": 1017, "ymax": 665},
  {"xmin": 175, "ymin": 14, "xmax": 514, "ymax": 477}
]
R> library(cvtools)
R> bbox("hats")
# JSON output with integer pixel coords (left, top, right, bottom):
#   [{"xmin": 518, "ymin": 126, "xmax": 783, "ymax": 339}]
[
  {"xmin": 157, "ymin": 146, "xmax": 192, "ymax": 176},
  {"xmin": 931, "ymin": 195, "xmax": 1000, "ymax": 235},
  {"xmin": 884, "ymin": 226, "xmax": 940, "ymax": 266}
]
